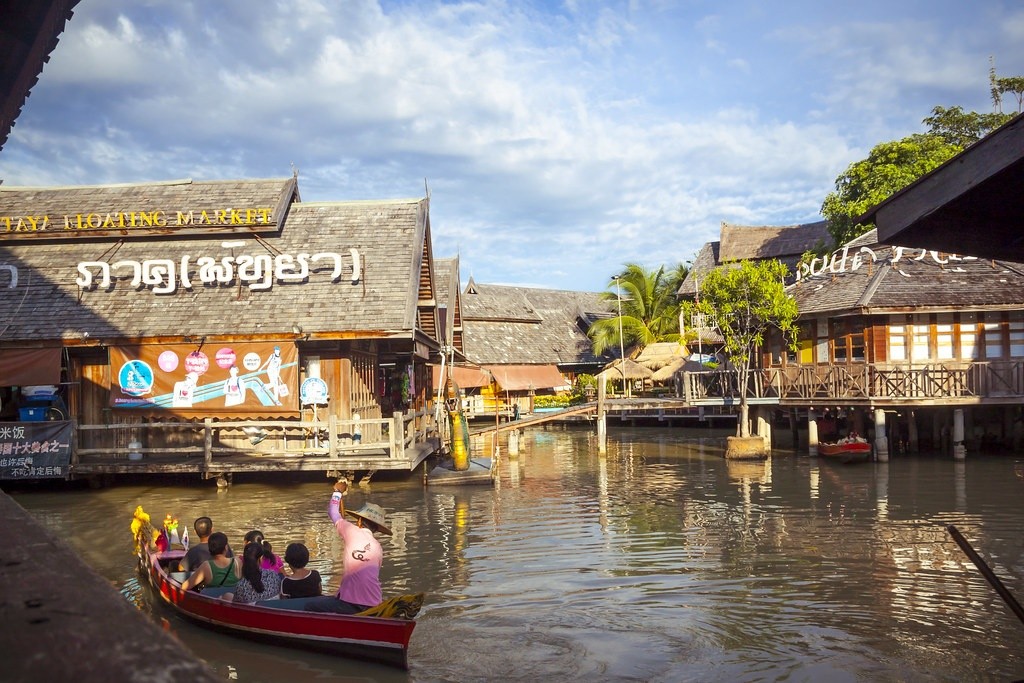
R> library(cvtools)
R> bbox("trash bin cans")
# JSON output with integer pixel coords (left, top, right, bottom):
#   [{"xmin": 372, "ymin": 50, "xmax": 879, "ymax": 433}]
[{"xmin": 18, "ymin": 407, "xmax": 48, "ymax": 421}]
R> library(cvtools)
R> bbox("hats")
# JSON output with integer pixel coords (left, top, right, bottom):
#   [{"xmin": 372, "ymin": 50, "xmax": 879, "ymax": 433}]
[{"xmin": 344, "ymin": 502, "xmax": 393, "ymax": 536}]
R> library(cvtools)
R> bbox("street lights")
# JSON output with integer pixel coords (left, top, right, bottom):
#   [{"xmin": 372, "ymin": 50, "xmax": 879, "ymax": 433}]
[
  {"xmin": 685, "ymin": 260, "xmax": 702, "ymax": 372},
  {"xmin": 610, "ymin": 274, "xmax": 628, "ymax": 396}
]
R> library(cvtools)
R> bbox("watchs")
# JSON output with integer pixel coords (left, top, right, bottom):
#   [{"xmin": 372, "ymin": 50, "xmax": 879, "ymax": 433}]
[{"xmin": 334, "ymin": 489, "xmax": 342, "ymax": 494}]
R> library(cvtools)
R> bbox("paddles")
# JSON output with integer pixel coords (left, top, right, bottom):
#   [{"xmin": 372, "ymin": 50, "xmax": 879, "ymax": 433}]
[
  {"xmin": 945, "ymin": 521, "xmax": 1024, "ymax": 625},
  {"xmin": 340, "ymin": 498, "xmax": 346, "ymax": 517}
]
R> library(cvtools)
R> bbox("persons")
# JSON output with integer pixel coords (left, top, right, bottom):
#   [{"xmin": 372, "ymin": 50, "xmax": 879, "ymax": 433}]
[
  {"xmin": 178, "ymin": 516, "xmax": 233, "ymax": 572},
  {"xmin": 238, "ymin": 530, "xmax": 287, "ymax": 577},
  {"xmin": 304, "ymin": 482, "xmax": 393, "ymax": 615},
  {"xmin": 280, "ymin": 543, "xmax": 322, "ymax": 599},
  {"xmin": 182, "ymin": 532, "xmax": 242, "ymax": 591},
  {"xmin": 222, "ymin": 542, "xmax": 281, "ymax": 603}
]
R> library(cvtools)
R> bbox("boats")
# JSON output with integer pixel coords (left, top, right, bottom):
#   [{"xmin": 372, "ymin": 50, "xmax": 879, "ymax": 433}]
[
  {"xmin": 816, "ymin": 440, "xmax": 874, "ymax": 462},
  {"xmin": 129, "ymin": 505, "xmax": 423, "ymax": 671}
]
